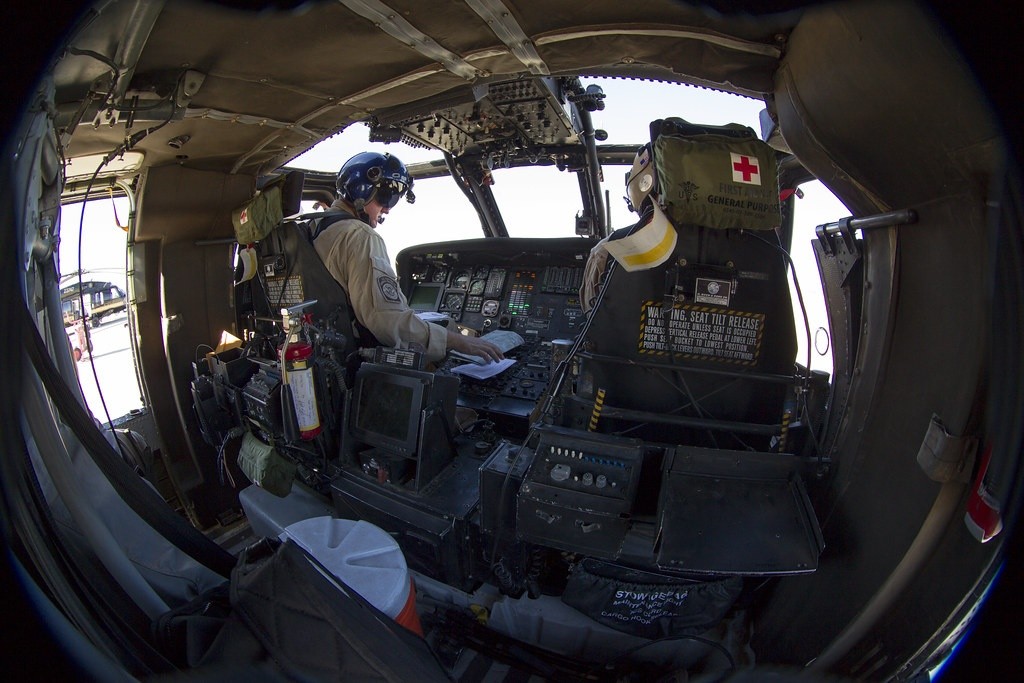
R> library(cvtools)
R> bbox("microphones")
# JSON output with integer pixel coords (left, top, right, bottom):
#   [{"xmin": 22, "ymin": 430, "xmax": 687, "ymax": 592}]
[{"xmin": 378, "ymin": 175, "xmax": 416, "ymax": 204}]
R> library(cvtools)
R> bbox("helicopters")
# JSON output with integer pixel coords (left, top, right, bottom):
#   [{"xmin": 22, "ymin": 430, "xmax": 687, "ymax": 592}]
[{"xmin": 58, "ymin": 266, "xmax": 127, "ymax": 336}]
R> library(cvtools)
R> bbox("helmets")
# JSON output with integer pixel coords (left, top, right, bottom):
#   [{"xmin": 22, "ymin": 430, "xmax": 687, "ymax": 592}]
[
  {"xmin": 335, "ymin": 152, "xmax": 415, "ymax": 208},
  {"xmin": 626, "ymin": 141, "xmax": 655, "ymax": 217}
]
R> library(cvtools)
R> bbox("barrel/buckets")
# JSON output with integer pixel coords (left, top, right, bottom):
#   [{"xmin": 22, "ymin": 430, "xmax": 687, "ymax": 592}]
[{"xmin": 273, "ymin": 517, "xmax": 426, "ymax": 645}]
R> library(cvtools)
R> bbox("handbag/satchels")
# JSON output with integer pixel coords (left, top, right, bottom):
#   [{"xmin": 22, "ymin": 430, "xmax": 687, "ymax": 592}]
[
  {"xmin": 236, "ymin": 434, "xmax": 295, "ymax": 497},
  {"xmin": 653, "ymin": 117, "xmax": 781, "ymax": 232},
  {"xmin": 151, "ymin": 536, "xmax": 450, "ymax": 682},
  {"xmin": 232, "ymin": 181, "xmax": 282, "ymax": 244}
]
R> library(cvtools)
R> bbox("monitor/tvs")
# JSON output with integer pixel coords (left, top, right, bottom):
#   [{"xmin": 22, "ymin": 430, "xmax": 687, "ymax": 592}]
[{"xmin": 349, "ymin": 371, "xmax": 427, "ymax": 456}]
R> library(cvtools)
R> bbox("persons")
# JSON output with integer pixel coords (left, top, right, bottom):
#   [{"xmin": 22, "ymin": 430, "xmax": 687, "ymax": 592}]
[
  {"xmin": 304, "ymin": 152, "xmax": 504, "ymax": 364},
  {"xmin": 578, "ymin": 142, "xmax": 656, "ymax": 318}
]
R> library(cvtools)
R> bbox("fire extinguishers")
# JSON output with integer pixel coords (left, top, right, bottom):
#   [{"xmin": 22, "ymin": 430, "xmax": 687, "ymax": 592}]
[{"xmin": 279, "ymin": 299, "xmax": 322, "ymax": 439}]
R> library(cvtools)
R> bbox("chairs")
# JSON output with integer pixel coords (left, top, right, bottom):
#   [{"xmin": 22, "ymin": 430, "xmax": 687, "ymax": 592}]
[
  {"xmin": 567, "ymin": 117, "xmax": 799, "ymax": 450},
  {"xmin": 230, "ymin": 171, "xmax": 356, "ymax": 360}
]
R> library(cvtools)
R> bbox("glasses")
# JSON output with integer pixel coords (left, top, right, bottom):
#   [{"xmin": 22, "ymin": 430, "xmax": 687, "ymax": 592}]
[{"xmin": 375, "ymin": 183, "xmax": 400, "ymax": 207}]
